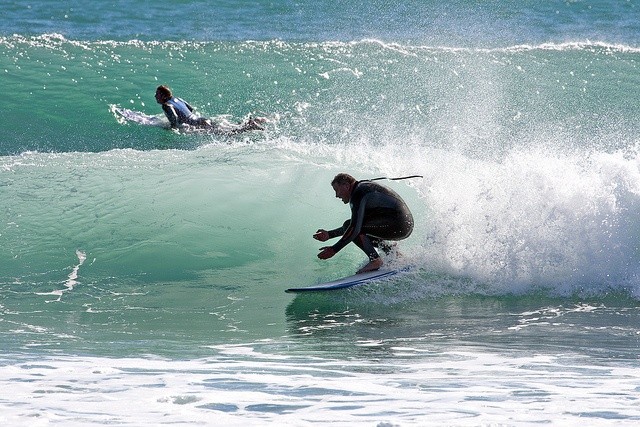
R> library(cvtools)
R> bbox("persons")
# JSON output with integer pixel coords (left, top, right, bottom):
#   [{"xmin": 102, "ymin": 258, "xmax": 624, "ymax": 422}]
[
  {"xmin": 312, "ymin": 172, "xmax": 423, "ymax": 274},
  {"xmin": 154, "ymin": 84, "xmax": 270, "ymax": 137}
]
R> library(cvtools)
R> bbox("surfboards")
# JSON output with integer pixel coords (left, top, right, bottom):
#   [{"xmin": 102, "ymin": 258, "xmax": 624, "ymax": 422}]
[
  {"xmin": 116, "ymin": 109, "xmax": 174, "ymax": 127},
  {"xmin": 284, "ymin": 260, "xmax": 418, "ymax": 292}
]
